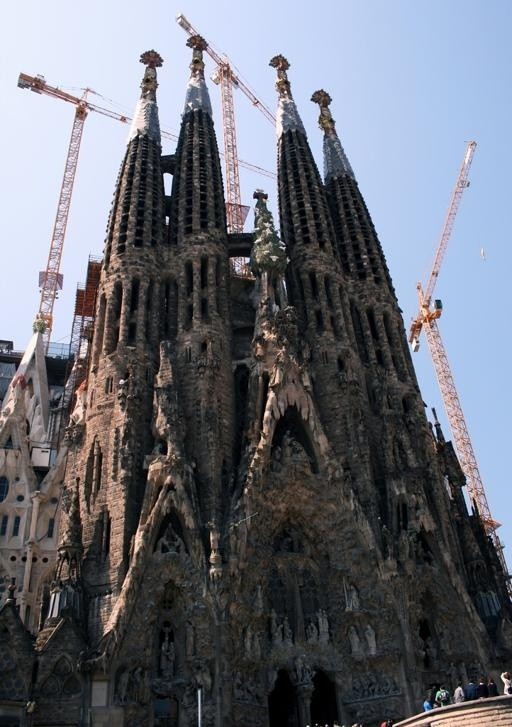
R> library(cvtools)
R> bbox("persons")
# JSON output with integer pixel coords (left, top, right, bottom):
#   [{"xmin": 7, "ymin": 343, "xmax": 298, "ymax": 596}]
[{"xmin": 304, "ymin": 668, "xmax": 511, "ymax": 726}]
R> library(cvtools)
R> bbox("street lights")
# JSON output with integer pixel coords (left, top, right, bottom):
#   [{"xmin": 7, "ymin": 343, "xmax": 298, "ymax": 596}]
[{"xmin": 193, "ymin": 679, "xmax": 204, "ymax": 727}]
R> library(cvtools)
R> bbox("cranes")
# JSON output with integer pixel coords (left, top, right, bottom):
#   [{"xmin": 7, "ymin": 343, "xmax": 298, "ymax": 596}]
[
  {"xmin": 176, "ymin": 11, "xmax": 279, "ymax": 275},
  {"xmin": 406, "ymin": 136, "xmax": 512, "ymax": 611},
  {"xmin": 11, "ymin": 70, "xmax": 279, "ymax": 357}
]
